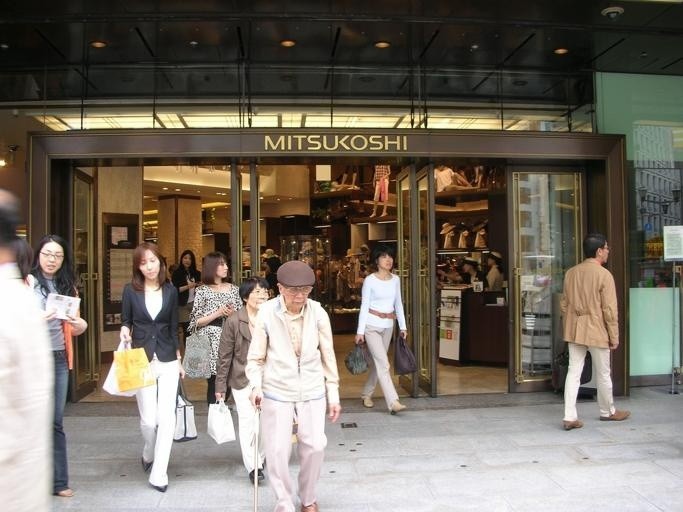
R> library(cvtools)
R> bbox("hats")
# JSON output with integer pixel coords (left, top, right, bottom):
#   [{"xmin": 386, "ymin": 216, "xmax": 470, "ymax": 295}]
[
  {"xmin": 277, "ymin": 260, "xmax": 315, "ymax": 287},
  {"xmin": 260, "ymin": 248, "xmax": 278, "ymax": 260},
  {"xmin": 439, "ymin": 222, "xmax": 455, "ymax": 235},
  {"xmin": 457, "ymin": 218, "xmax": 489, "ymax": 233},
  {"xmin": 344, "ymin": 248, "xmax": 365, "ymax": 259}
]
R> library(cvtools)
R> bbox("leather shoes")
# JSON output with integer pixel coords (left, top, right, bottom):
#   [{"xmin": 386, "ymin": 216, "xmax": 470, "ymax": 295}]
[{"xmin": 248, "ymin": 465, "xmax": 266, "ymax": 485}]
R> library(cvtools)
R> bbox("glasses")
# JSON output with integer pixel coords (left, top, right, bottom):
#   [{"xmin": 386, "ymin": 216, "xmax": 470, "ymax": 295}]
[
  {"xmin": 284, "ymin": 285, "xmax": 313, "ymax": 295},
  {"xmin": 40, "ymin": 251, "xmax": 64, "ymax": 260}
]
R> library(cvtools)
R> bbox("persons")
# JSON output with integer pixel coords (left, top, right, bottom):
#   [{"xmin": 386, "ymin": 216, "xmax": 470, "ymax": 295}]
[
  {"xmin": 433, "ymin": 160, "xmax": 480, "ymax": 192},
  {"xmin": 355, "ymin": 245, "xmax": 408, "ymax": 414},
  {"xmin": 370, "ymin": 164, "xmax": 391, "ymax": 217},
  {"xmin": 0, "ymin": 189, "xmax": 55, "ymax": 512},
  {"xmin": 438, "ymin": 258, "xmax": 488, "ymax": 291},
  {"xmin": 561, "ymin": 232, "xmax": 631, "ymax": 430},
  {"xmin": 485, "ymin": 251, "xmax": 504, "ymax": 294},
  {"xmin": 25, "ymin": 236, "xmax": 88, "ymax": 497},
  {"xmin": 338, "ymin": 167, "xmax": 358, "ymax": 191}
]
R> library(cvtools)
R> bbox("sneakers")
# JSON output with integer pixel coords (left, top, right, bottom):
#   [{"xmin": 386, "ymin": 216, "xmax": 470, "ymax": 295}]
[
  {"xmin": 600, "ymin": 410, "xmax": 631, "ymax": 421},
  {"xmin": 140, "ymin": 457, "xmax": 168, "ymax": 492},
  {"xmin": 54, "ymin": 487, "xmax": 73, "ymax": 499},
  {"xmin": 561, "ymin": 416, "xmax": 586, "ymax": 432},
  {"xmin": 299, "ymin": 503, "xmax": 319, "ymax": 512}
]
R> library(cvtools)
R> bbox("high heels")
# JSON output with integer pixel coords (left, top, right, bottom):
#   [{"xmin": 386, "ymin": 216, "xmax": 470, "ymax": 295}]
[
  {"xmin": 360, "ymin": 396, "xmax": 374, "ymax": 408},
  {"xmin": 390, "ymin": 400, "xmax": 407, "ymax": 415}
]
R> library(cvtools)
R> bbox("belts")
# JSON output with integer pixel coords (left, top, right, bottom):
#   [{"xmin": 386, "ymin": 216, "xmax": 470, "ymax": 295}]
[{"xmin": 368, "ymin": 308, "xmax": 398, "ymax": 321}]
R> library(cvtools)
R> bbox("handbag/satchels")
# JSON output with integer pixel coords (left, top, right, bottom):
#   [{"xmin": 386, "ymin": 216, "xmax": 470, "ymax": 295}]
[
  {"xmin": 344, "ymin": 340, "xmax": 369, "ymax": 377},
  {"xmin": 205, "ymin": 396, "xmax": 237, "ymax": 445},
  {"xmin": 182, "ymin": 316, "xmax": 213, "ymax": 380},
  {"xmin": 393, "ymin": 329, "xmax": 419, "ymax": 376},
  {"xmin": 101, "ymin": 339, "xmax": 159, "ymax": 398},
  {"xmin": 173, "ymin": 374, "xmax": 198, "ymax": 443}
]
